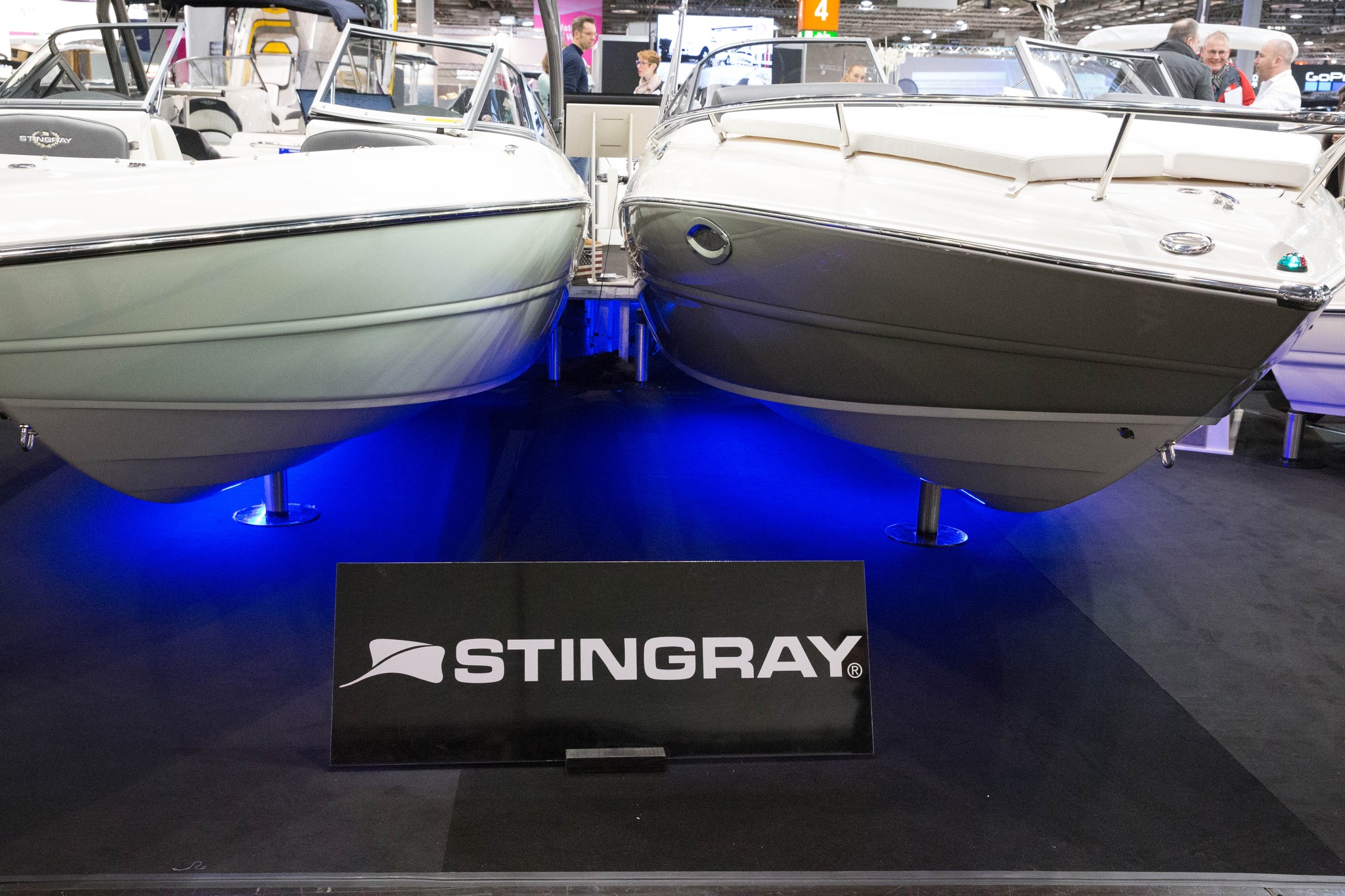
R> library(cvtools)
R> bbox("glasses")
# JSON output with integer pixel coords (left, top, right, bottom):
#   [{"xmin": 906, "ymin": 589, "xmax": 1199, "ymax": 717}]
[
  {"xmin": 581, "ymin": 31, "xmax": 597, "ymax": 37},
  {"xmin": 636, "ymin": 61, "xmax": 654, "ymax": 66},
  {"xmin": 1204, "ymin": 47, "xmax": 1230, "ymax": 57}
]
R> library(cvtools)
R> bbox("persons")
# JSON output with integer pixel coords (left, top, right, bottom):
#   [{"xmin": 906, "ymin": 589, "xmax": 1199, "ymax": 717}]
[
  {"xmin": 1108, "ymin": 18, "xmax": 1301, "ymax": 111},
  {"xmin": 538, "ymin": 16, "xmax": 602, "ymax": 248},
  {"xmin": 841, "ymin": 65, "xmax": 865, "ymax": 82},
  {"xmin": 1321, "ymin": 83, "xmax": 1345, "ymax": 211},
  {"xmin": 620, "ymin": 49, "xmax": 678, "ymax": 249}
]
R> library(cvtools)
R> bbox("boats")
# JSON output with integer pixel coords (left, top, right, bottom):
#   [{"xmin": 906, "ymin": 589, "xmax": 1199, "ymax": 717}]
[
  {"xmin": 615, "ymin": 1, "xmax": 1341, "ymax": 512},
  {"xmin": 0, "ymin": 1, "xmax": 594, "ymax": 504}
]
